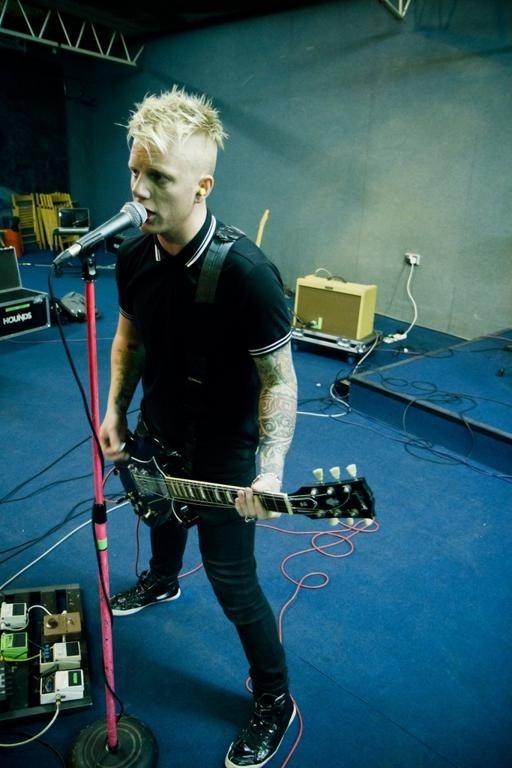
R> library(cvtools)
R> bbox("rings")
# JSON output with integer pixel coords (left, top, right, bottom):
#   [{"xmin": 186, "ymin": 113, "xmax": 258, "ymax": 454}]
[{"xmin": 243, "ymin": 515, "xmax": 256, "ymax": 523}]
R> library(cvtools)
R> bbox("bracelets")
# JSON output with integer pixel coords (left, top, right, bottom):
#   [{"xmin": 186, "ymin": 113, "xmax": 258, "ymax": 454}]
[{"xmin": 252, "ymin": 470, "xmax": 283, "ymax": 487}]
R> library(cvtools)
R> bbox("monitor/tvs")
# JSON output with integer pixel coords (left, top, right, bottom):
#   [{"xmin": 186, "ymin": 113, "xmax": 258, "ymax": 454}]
[{"xmin": 57, "ymin": 207, "xmax": 91, "ymax": 233}]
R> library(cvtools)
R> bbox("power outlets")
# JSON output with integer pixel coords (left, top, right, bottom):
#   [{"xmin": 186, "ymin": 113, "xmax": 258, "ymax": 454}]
[{"xmin": 404, "ymin": 253, "xmax": 419, "ymax": 266}]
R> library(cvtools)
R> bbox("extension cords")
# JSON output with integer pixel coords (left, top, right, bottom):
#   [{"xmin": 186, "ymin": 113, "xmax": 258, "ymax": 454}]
[{"xmin": 383, "ymin": 332, "xmax": 407, "ymax": 344}]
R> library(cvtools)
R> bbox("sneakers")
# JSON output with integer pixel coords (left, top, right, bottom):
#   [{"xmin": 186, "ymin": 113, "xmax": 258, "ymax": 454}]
[
  {"xmin": 108, "ymin": 569, "xmax": 182, "ymax": 617},
  {"xmin": 223, "ymin": 690, "xmax": 297, "ymax": 768}
]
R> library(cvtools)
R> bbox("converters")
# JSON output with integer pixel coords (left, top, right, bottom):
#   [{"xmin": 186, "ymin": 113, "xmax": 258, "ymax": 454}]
[{"xmin": 334, "ymin": 377, "xmax": 351, "ymax": 397}]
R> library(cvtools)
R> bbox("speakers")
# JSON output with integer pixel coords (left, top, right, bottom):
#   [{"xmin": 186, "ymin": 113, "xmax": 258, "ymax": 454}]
[{"xmin": 291, "ymin": 275, "xmax": 377, "ymax": 340}]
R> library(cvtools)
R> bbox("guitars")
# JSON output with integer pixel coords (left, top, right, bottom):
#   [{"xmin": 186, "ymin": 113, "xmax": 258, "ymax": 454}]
[{"xmin": 114, "ymin": 430, "xmax": 376, "ymax": 530}]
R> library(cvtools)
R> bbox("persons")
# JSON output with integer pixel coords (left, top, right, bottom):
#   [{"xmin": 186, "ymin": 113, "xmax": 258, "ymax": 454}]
[{"xmin": 100, "ymin": 87, "xmax": 299, "ymax": 765}]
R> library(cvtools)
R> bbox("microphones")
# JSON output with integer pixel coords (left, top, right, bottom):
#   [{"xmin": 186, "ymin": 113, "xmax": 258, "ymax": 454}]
[
  {"xmin": 52, "ymin": 201, "xmax": 147, "ymax": 269},
  {"xmin": 304, "ymin": 320, "xmax": 316, "ymax": 328}
]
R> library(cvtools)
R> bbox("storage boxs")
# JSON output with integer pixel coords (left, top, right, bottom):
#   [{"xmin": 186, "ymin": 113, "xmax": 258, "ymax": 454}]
[{"xmin": 0, "ymin": 246, "xmax": 51, "ymax": 340}]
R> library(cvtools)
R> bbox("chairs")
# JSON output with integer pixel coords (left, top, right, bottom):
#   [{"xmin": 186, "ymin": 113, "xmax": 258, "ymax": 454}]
[{"xmin": 10, "ymin": 192, "xmax": 90, "ymax": 277}]
